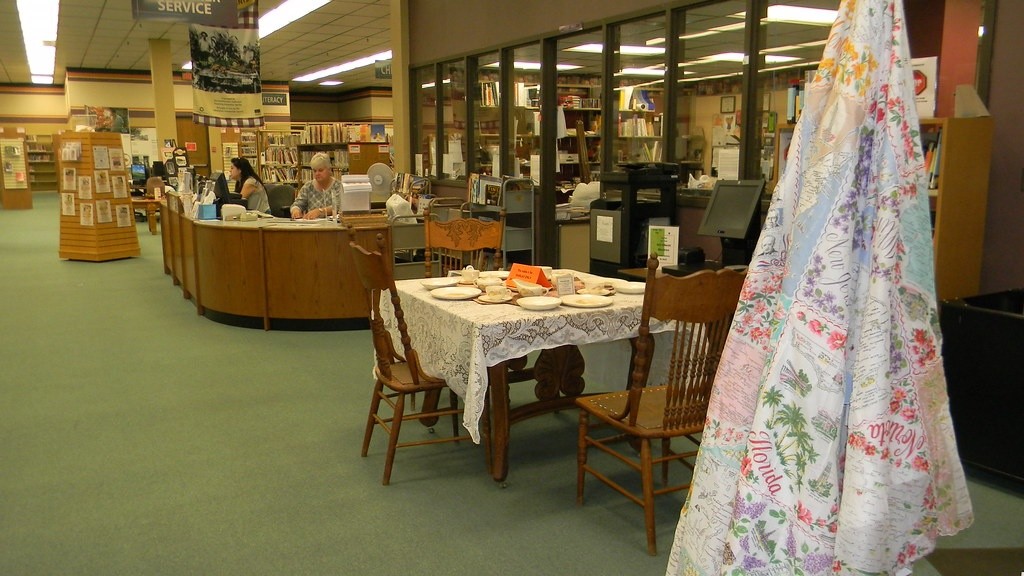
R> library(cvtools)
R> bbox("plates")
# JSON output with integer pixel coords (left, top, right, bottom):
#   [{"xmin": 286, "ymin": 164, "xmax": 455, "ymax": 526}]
[
  {"xmin": 516, "ymin": 296, "xmax": 561, "ymax": 310},
  {"xmin": 419, "ymin": 277, "xmax": 460, "ymax": 290},
  {"xmin": 478, "ymin": 295, "xmax": 512, "ymax": 303},
  {"xmin": 553, "ymin": 269, "xmax": 646, "ymax": 307},
  {"xmin": 479, "ymin": 270, "xmax": 510, "ymax": 280},
  {"xmin": 430, "ymin": 286, "xmax": 482, "ymax": 300}
]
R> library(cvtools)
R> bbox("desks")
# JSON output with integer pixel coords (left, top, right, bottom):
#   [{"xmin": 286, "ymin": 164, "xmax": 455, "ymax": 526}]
[
  {"xmin": 373, "ymin": 268, "xmax": 710, "ymax": 483},
  {"xmin": 130, "ymin": 196, "xmax": 159, "ymax": 235}
]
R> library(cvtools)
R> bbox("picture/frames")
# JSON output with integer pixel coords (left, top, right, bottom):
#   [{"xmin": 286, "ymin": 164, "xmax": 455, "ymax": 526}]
[
  {"xmin": 85, "ymin": 105, "xmax": 132, "ymax": 136},
  {"xmin": 773, "ymin": 124, "xmax": 796, "ymax": 189},
  {"xmin": 720, "ymin": 96, "xmax": 736, "ymax": 113}
]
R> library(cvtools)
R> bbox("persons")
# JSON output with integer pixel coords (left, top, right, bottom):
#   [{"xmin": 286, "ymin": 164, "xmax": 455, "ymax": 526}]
[
  {"xmin": 290, "ymin": 153, "xmax": 344, "ymax": 220},
  {"xmin": 229, "ymin": 158, "xmax": 272, "ymax": 215},
  {"xmin": 199, "ymin": 30, "xmax": 252, "ymax": 91}
]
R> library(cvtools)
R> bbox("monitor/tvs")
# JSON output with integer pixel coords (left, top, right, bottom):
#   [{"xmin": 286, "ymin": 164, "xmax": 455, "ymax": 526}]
[
  {"xmin": 131, "ymin": 164, "xmax": 146, "ymax": 183},
  {"xmin": 696, "ymin": 179, "xmax": 766, "ymax": 241},
  {"xmin": 181, "ymin": 167, "xmax": 232, "ymax": 205}
]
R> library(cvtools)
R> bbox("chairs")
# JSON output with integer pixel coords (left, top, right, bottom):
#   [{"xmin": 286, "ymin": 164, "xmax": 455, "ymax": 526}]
[
  {"xmin": 267, "ymin": 184, "xmax": 296, "ymax": 219},
  {"xmin": 574, "ymin": 252, "xmax": 750, "ymax": 552},
  {"xmin": 346, "ymin": 240, "xmax": 495, "ymax": 485},
  {"xmin": 420, "ymin": 206, "xmax": 503, "ymax": 278}
]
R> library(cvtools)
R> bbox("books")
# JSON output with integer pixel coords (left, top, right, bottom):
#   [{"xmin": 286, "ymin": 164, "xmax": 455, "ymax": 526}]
[
  {"xmin": 618, "ymin": 112, "xmax": 654, "ymax": 136},
  {"xmin": 485, "ymin": 83, "xmax": 498, "ymax": 105},
  {"xmin": 642, "ymin": 141, "xmax": 662, "ymax": 161},
  {"xmin": 261, "ymin": 122, "xmax": 386, "ymax": 182}
]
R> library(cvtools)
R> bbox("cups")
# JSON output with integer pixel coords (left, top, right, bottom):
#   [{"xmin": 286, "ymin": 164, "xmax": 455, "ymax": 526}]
[
  {"xmin": 473, "ymin": 277, "xmax": 505, "ymax": 291},
  {"xmin": 513, "ymin": 279, "xmax": 549, "ymax": 296},
  {"xmin": 460, "ymin": 265, "xmax": 480, "ymax": 285},
  {"xmin": 537, "ymin": 266, "xmax": 553, "ymax": 281},
  {"xmin": 485, "ymin": 286, "xmax": 512, "ymax": 300}
]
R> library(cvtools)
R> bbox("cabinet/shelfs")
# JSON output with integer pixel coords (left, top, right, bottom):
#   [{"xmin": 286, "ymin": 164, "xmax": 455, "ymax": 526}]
[
  {"xmin": 918, "ymin": 118, "xmax": 993, "ymax": 301},
  {"xmin": 476, "ymin": 86, "xmax": 663, "ymax": 182},
  {"xmin": 25, "ymin": 134, "xmax": 59, "ymax": 191},
  {"xmin": 257, "ymin": 130, "xmax": 300, "ymax": 187}
]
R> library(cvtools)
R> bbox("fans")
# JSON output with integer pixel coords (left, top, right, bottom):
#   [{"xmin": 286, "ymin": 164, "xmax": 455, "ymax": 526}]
[{"xmin": 366, "ymin": 163, "xmax": 393, "ymax": 197}]
